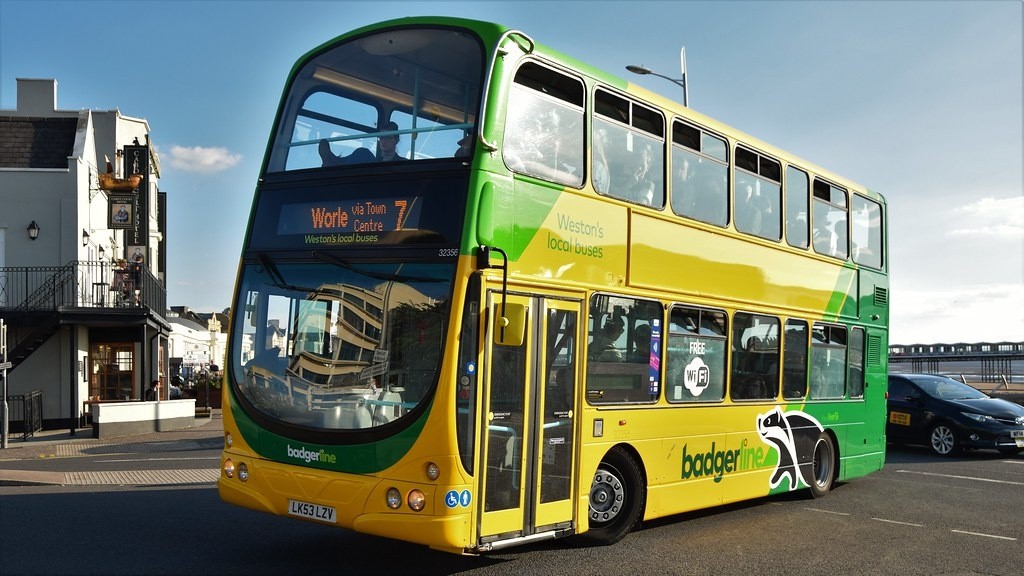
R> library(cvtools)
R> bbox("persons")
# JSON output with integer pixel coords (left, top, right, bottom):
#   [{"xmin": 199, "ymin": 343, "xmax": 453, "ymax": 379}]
[
  {"xmin": 491, "ymin": 118, "xmax": 859, "ymax": 263},
  {"xmin": 556, "ymin": 311, "xmax": 653, "ymax": 409},
  {"xmin": 669, "ymin": 334, "xmax": 822, "ymax": 398},
  {"xmin": 318, "ymin": 122, "xmax": 408, "ymax": 168},
  {"xmin": 112, "ymin": 255, "xmax": 146, "ymax": 305},
  {"xmin": 210, "ymin": 359, "xmax": 219, "ymax": 372},
  {"xmin": 145, "ymin": 380, "xmax": 161, "ymax": 401}
]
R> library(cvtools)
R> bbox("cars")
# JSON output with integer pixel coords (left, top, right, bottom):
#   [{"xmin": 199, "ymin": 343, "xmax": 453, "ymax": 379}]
[{"xmin": 884, "ymin": 373, "xmax": 1024, "ymax": 455}]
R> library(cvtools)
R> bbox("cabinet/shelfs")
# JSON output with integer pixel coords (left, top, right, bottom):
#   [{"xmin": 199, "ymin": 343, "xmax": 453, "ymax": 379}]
[{"xmin": 82, "ymin": 399, "xmax": 140, "ymax": 427}]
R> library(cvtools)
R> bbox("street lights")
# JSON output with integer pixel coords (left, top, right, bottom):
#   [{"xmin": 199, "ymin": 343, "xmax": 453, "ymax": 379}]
[{"xmin": 624, "ymin": 46, "xmax": 689, "ymax": 107}]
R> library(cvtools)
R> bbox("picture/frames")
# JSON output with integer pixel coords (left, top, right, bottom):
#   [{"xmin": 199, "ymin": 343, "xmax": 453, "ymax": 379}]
[{"xmin": 84, "ymin": 355, "xmax": 88, "ymax": 383}]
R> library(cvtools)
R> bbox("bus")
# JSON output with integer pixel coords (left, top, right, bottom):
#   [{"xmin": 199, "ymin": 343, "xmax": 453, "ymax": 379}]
[{"xmin": 216, "ymin": 16, "xmax": 893, "ymax": 559}]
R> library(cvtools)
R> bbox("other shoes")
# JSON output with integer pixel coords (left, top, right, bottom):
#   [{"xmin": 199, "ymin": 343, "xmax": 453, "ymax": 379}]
[{"xmin": 319, "ymin": 139, "xmax": 340, "ymax": 167}]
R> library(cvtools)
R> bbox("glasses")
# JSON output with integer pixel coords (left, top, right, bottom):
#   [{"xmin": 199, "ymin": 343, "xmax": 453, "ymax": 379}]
[{"xmin": 377, "ymin": 137, "xmax": 397, "ymax": 142}]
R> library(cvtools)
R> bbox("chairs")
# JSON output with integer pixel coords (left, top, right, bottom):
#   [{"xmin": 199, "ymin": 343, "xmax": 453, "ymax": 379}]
[{"xmin": 574, "ymin": 124, "xmax": 872, "ymax": 265}]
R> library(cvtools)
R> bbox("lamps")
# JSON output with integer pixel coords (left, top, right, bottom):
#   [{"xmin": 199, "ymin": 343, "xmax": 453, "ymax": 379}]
[
  {"xmin": 26, "ymin": 220, "xmax": 40, "ymax": 240},
  {"xmin": 99, "ymin": 244, "xmax": 105, "ymax": 259},
  {"xmin": 83, "ymin": 231, "xmax": 90, "ymax": 246}
]
